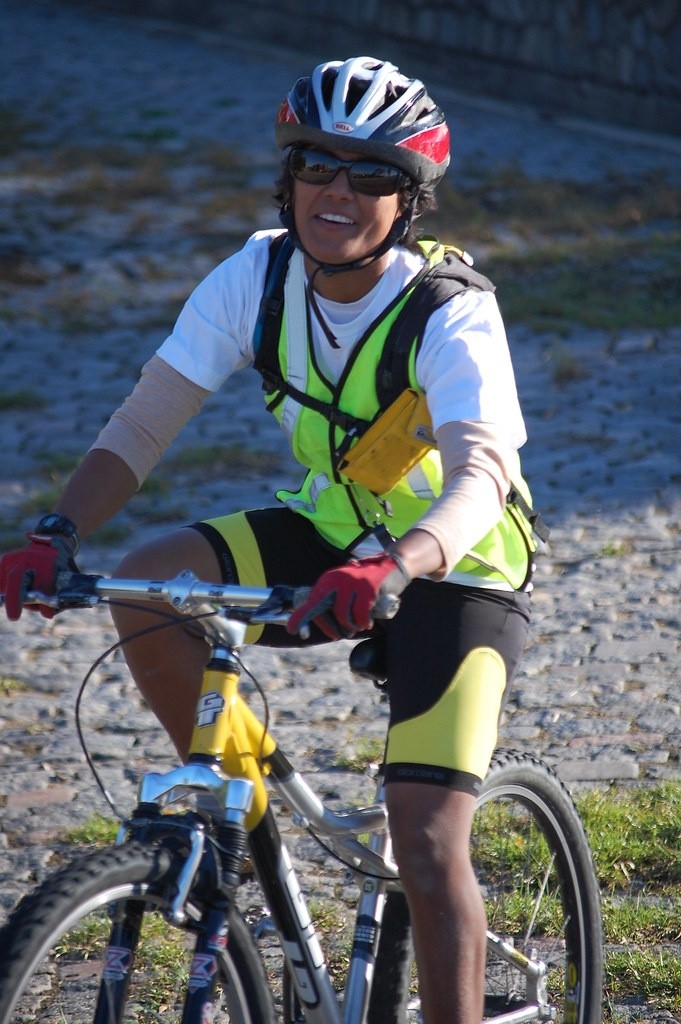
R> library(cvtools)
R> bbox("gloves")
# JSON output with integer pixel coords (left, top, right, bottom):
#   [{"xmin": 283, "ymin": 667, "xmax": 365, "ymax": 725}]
[
  {"xmin": 1, "ymin": 533, "xmax": 79, "ymax": 623},
  {"xmin": 284, "ymin": 552, "xmax": 415, "ymax": 642}
]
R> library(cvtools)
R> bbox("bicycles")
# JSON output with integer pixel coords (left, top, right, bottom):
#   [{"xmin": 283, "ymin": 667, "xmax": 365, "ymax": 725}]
[{"xmin": 0, "ymin": 570, "xmax": 602, "ymax": 1024}]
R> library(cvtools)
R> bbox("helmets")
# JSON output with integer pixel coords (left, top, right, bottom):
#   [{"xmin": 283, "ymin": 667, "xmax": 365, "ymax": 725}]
[{"xmin": 275, "ymin": 57, "xmax": 451, "ymax": 192}]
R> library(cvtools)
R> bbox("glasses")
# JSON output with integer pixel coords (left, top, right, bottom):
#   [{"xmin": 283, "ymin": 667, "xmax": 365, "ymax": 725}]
[{"xmin": 288, "ymin": 148, "xmax": 406, "ymax": 196}]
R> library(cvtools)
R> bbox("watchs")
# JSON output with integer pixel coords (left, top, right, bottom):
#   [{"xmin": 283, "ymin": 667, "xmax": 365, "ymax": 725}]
[{"xmin": 34, "ymin": 515, "xmax": 78, "ymax": 558}]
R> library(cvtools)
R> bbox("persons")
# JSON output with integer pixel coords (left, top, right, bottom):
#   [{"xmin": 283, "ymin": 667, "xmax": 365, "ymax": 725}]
[{"xmin": 0, "ymin": 56, "xmax": 538, "ymax": 1024}]
